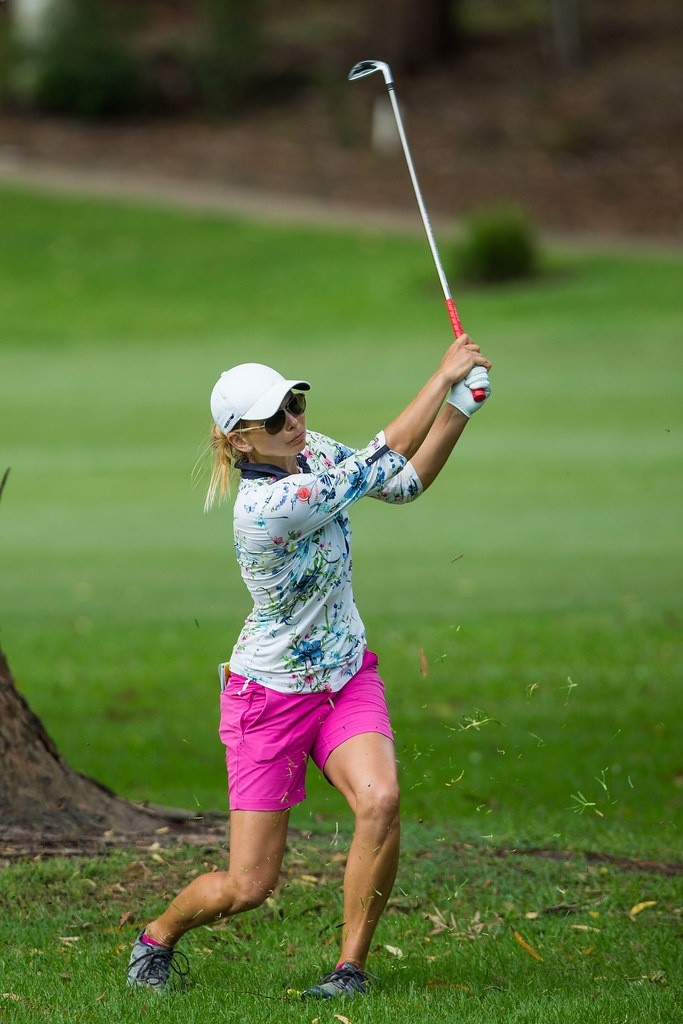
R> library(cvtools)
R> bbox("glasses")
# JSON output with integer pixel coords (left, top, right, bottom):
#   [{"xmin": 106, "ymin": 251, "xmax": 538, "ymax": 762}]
[{"xmin": 233, "ymin": 392, "xmax": 306, "ymax": 435}]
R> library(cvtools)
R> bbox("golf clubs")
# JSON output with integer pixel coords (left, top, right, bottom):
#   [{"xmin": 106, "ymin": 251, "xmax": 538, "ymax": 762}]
[{"xmin": 348, "ymin": 57, "xmax": 489, "ymax": 402}]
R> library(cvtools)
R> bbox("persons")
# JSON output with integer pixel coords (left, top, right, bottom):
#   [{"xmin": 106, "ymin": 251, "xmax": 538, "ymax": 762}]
[{"xmin": 127, "ymin": 333, "xmax": 490, "ymax": 1004}]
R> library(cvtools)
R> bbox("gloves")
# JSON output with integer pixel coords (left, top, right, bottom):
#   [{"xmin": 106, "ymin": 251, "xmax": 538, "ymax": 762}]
[{"xmin": 446, "ymin": 366, "xmax": 492, "ymax": 418}]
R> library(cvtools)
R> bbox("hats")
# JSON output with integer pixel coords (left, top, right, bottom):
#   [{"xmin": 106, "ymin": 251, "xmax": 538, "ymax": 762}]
[{"xmin": 210, "ymin": 363, "xmax": 312, "ymax": 437}]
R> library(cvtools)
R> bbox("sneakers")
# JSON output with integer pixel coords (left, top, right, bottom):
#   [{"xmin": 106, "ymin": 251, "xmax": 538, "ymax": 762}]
[
  {"xmin": 301, "ymin": 962, "xmax": 371, "ymax": 999},
  {"xmin": 126, "ymin": 925, "xmax": 190, "ymax": 988}
]
